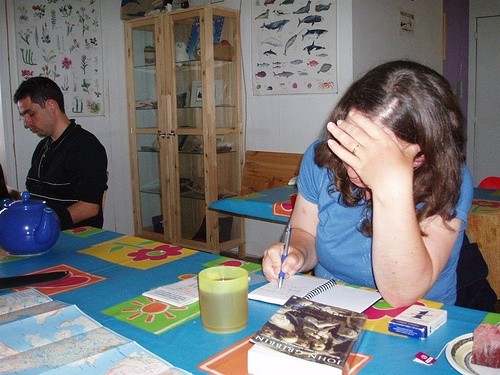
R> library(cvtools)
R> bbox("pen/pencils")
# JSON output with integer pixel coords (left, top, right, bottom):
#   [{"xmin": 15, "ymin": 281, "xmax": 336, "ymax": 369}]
[{"xmin": 278, "ymin": 224, "xmax": 293, "ymax": 290}]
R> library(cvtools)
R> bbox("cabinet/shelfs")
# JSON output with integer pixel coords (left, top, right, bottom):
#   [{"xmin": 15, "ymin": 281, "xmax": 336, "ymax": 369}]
[{"xmin": 124, "ymin": 4, "xmax": 246, "ymax": 260}]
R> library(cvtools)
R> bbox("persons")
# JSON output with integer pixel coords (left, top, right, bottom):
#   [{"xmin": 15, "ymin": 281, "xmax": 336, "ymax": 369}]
[
  {"xmin": 4, "ymin": 76, "xmax": 108, "ymax": 230},
  {"xmin": 262, "ymin": 61, "xmax": 474, "ymax": 306}
]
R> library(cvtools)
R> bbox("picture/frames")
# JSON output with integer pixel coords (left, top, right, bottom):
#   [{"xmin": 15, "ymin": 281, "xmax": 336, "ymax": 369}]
[{"xmin": 190, "ymin": 81, "xmax": 223, "ymax": 107}]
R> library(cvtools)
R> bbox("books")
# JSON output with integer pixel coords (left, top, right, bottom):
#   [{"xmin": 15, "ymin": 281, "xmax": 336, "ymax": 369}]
[{"xmin": 248, "ymin": 274, "xmax": 385, "ymax": 375}]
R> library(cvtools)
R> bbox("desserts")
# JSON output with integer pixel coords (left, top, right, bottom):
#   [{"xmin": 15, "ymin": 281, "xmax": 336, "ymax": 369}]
[{"xmin": 471, "ymin": 324, "xmax": 500, "ymax": 369}]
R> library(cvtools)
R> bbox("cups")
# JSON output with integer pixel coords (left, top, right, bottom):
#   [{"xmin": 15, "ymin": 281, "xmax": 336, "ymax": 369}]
[{"xmin": 199, "ymin": 266, "xmax": 249, "ymax": 334}]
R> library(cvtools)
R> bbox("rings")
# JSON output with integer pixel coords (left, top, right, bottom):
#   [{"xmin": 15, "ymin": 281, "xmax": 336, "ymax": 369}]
[{"xmin": 352, "ymin": 145, "xmax": 362, "ymax": 154}]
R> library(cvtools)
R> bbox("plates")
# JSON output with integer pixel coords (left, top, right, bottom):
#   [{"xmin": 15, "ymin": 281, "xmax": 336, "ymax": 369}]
[{"xmin": 445, "ymin": 331, "xmax": 500, "ymax": 375}]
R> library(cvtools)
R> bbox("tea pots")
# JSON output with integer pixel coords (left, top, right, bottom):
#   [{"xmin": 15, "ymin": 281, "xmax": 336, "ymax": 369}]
[{"xmin": 0, "ymin": 190, "xmax": 61, "ymax": 254}]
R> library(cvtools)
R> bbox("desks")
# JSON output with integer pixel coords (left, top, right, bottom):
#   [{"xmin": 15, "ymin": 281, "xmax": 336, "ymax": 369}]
[
  {"xmin": 0, "ymin": 227, "xmax": 500, "ymax": 375},
  {"xmin": 208, "ymin": 183, "xmax": 500, "ymax": 244}
]
